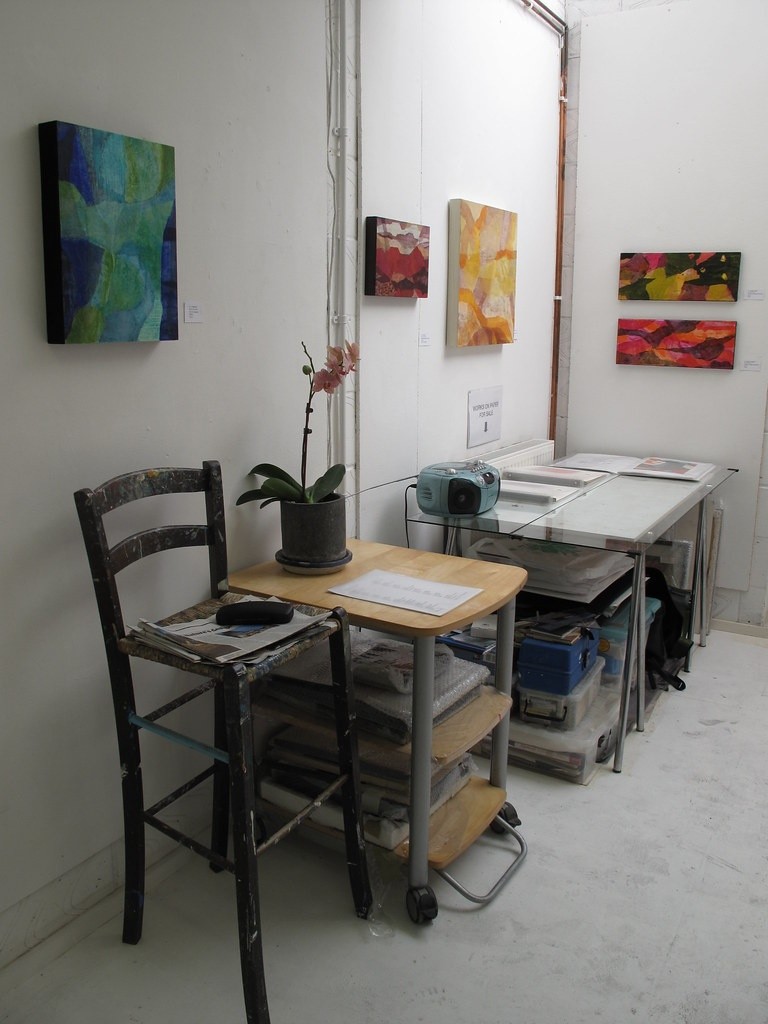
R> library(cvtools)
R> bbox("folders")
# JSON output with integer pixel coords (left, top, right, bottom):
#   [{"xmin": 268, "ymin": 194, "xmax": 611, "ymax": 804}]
[{"xmin": 502, "ymin": 465, "xmax": 608, "ymax": 488}]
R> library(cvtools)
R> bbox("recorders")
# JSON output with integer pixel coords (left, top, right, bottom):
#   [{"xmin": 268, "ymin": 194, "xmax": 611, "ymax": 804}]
[{"xmin": 417, "ymin": 460, "xmax": 501, "ymax": 518}]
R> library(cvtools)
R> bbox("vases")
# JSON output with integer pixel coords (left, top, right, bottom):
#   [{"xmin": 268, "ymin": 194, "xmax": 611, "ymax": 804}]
[{"xmin": 275, "ymin": 493, "xmax": 353, "ymax": 575}]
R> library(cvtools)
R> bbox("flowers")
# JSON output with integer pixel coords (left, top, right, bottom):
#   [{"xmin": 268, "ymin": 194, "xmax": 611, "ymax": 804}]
[{"xmin": 236, "ymin": 337, "xmax": 363, "ymax": 510}]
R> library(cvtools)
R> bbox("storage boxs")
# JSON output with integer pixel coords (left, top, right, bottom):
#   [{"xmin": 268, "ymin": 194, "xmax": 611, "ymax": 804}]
[{"xmin": 435, "ymin": 597, "xmax": 661, "ymax": 785}]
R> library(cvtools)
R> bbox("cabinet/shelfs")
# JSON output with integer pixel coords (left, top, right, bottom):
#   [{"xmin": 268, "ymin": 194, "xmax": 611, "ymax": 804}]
[
  {"xmin": 226, "ymin": 537, "xmax": 529, "ymax": 924},
  {"xmin": 455, "ymin": 438, "xmax": 555, "ymax": 481}
]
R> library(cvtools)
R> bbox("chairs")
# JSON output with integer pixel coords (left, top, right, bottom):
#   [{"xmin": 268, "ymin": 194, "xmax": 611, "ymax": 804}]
[{"xmin": 70, "ymin": 459, "xmax": 375, "ymax": 1024}]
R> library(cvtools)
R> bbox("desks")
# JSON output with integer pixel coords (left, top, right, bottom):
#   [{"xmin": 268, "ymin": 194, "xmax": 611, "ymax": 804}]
[{"xmin": 406, "ymin": 453, "xmax": 740, "ymax": 774}]
